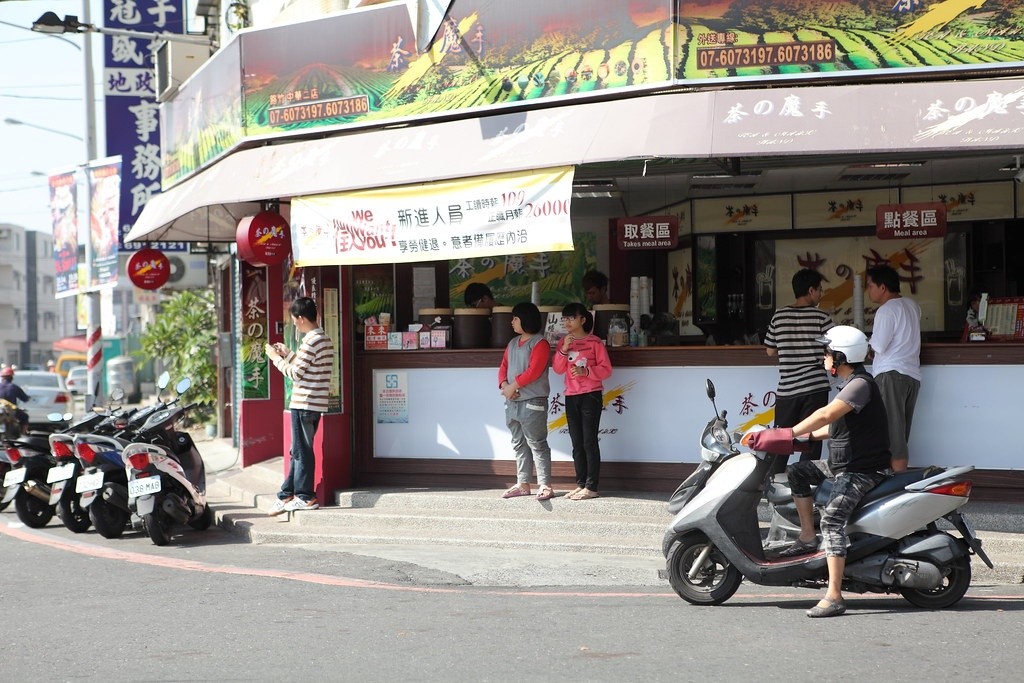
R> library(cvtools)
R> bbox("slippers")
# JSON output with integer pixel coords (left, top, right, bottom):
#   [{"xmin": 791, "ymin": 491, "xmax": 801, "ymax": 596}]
[
  {"xmin": 571, "ymin": 491, "xmax": 600, "ymax": 502},
  {"xmin": 564, "ymin": 487, "xmax": 580, "ymax": 500},
  {"xmin": 502, "ymin": 483, "xmax": 531, "ymax": 499},
  {"xmin": 536, "ymin": 485, "xmax": 554, "ymax": 500}
]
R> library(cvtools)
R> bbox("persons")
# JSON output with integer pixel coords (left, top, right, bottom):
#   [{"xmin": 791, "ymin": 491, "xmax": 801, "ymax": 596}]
[
  {"xmin": 863, "ymin": 263, "xmax": 920, "ymax": 471},
  {"xmin": 779, "ymin": 325, "xmax": 893, "ymax": 617},
  {"xmin": 265, "ymin": 297, "xmax": 333, "ymax": 516},
  {"xmin": 764, "ymin": 268, "xmax": 835, "ymax": 474},
  {"xmin": 552, "ymin": 303, "xmax": 612, "ymax": 500},
  {"xmin": 465, "ymin": 284, "xmax": 503, "ymax": 317},
  {"xmin": 583, "ymin": 270, "xmax": 614, "ymax": 339},
  {"xmin": 0, "ymin": 366, "xmax": 30, "ymax": 433},
  {"xmin": 499, "ymin": 302, "xmax": 553, "ymax": 500}
]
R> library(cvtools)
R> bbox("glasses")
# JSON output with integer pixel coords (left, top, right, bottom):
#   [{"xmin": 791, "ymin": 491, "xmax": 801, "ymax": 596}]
[{"xmin": 561, "ymin": 315, "xmax": 584, "ymax": 322}]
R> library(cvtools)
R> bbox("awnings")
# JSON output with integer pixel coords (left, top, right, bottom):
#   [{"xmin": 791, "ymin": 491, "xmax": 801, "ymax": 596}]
[{"xmin": 124, "ymin": 78, "xmax": 1024, "ymax": 243}]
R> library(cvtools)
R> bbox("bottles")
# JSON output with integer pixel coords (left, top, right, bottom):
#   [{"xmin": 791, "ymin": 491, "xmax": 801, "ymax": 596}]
[
  {"xmin": 606, "ymin": 318, "xmax": 629, "ymax": 346},
  {"xmin": 727, "ymin": 294, "xmax": 746, "ymax": 319}
]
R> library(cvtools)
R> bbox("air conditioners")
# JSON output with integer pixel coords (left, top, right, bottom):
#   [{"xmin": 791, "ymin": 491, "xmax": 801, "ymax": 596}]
[{"xmin": 154, "ymin": 35, "xmax": 208, "ymax": 103}]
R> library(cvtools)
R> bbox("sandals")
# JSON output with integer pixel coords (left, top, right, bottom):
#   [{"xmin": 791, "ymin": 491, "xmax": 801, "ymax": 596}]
[
  {"xmin": 284, "ymin": 495, "xmax": 319, "ymax": 511},
  {"xmin": 268, "ymin": 495, "xmax": 293, "ymax": 516}
]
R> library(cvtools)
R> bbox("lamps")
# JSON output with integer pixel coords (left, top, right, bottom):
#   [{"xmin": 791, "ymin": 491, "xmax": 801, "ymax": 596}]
[
  {"xmin": 31, "ymin": 12, "xmax": 211, "ymax": 48},
  {"xmin": 1014, "ymin": 155, "xmax": 1024, "ymax": 184}
]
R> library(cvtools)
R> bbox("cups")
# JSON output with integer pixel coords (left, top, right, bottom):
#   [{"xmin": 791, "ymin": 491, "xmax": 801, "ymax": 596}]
[
  {"xmin": 531, "ymin": 282, "xmax": 540, "ymax": 306},
  {"xmin": 851, "ymin": 275, "xmax": 865, "ymax": 334},
  {"xmin": 638, "ymin": 334, "xmax": 647, "ymax": 347},
  {"xmin": 629, "ymin": 333, "xmax": 638, "ymax": 347},
  {"xmin": 629, "ymin": 276, "xmax": 649, "ymax": 334}
]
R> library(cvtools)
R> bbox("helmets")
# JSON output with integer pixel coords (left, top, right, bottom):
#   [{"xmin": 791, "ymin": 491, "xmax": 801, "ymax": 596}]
[
  {"xmin": 814, "ymin": 324, "xmax": 871, "ymax": 365},
  {"xmin": 0, "ymin": 367, "xmax": 14, "ymax": 378}
]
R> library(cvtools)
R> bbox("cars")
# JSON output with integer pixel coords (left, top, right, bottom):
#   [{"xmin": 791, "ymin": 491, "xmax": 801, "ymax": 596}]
[
  {"xmin": 63, "ymin": 366, "xmax": 88, "ymax": 396},
  {"xmin": 11, "ymin": 371, "xmax": 79, "ymax": 432}
]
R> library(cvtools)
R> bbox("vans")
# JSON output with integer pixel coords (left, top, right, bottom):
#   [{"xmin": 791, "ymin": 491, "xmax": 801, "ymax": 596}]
[{"xmin": 47, "ymin": 353, "xmax": 87, "ymax": 381}]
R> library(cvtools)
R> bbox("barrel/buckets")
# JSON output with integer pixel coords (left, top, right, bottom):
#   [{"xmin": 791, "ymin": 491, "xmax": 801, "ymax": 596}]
[
  {"xmin": 418, "ymin": 308, "xmax": 452, "ymax": 331},
  {"xmin": 453, "ymin": 308, "xmax": 491, "ymax": 348},
  {"xmin": 492, "ymin": 306, "xmax": 519, "ymax": 349},
  {"xmin": 537, "ymin": 306, "xmax": 564, "ymax": 337}
]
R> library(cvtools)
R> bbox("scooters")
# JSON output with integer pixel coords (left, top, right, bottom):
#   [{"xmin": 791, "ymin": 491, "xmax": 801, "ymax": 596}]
[
  {"xmin": 115, "ymin": 377, "xmax": 213, "ymax": 546},
  {"xmin": 0, "ymin": 370, "xmax": 180, "ymax": 539},
  {"xmin": 662, "ymin": 423, "xmax": 993, "ymax": 610},
  {"xmin": 661, "ymin": 377, "xmax": 822, "ymax": 559}
]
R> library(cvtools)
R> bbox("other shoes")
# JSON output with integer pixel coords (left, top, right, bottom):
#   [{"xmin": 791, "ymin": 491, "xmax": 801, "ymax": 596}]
[
  {"xmin": 779, "ymin": 535, "xmax": 821, "ymax": 558},
  {"xmin": 806, "ymin": 597, "xmax": 847, "ymax": 618}
]
